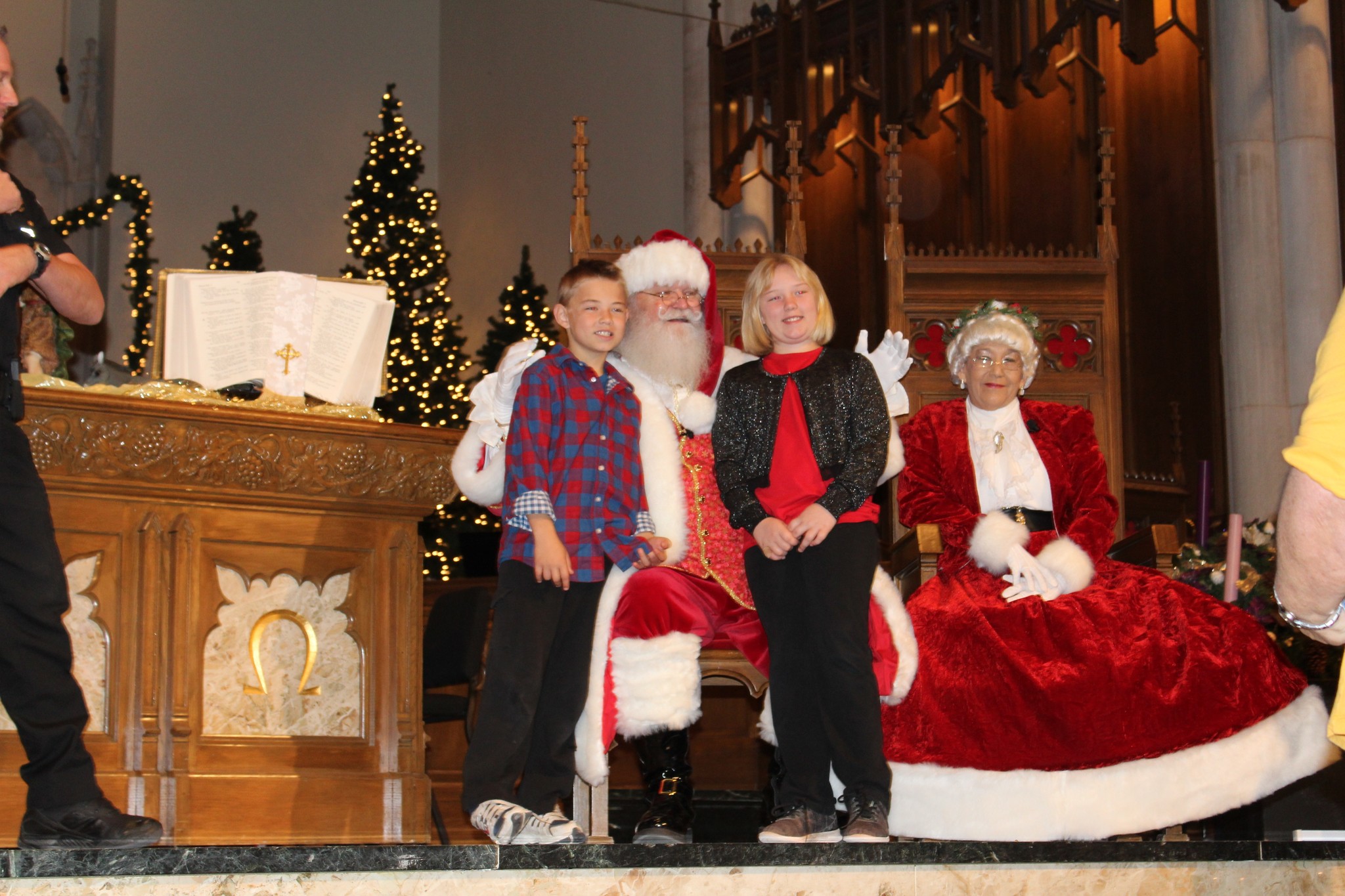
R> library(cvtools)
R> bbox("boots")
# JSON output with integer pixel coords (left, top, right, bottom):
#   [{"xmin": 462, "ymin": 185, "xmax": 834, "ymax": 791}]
[
  {"xmin": 758, "ymin": 746, "xmax": 787, "ymax": 833},
  {"xmin": 632, "ymin": 727, "xmax": 694, "ymax": 844}
]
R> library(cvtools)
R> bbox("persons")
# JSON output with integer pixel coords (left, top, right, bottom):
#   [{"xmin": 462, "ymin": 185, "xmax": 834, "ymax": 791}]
[
  {"xmin": 461, "ymin": 258, "xmax": 669, "ymax": 845},
  {"xmin": 1272, "ymin": 283, "xmax": 1345, "ymax": 753},
  {"xmin": 0, "ymin": 20, "xmax": 169, "ymax": 850},
  {"xmin": 711, "ymin": 250, "xmax": 895, "ymax": 845},
  {"xmin": 449, "ymin": 226, "xmax": 912, "ymax": 850},
  {"xmin": 877, "ymin": 293, "xmax": 1192, "ymax": 841}
]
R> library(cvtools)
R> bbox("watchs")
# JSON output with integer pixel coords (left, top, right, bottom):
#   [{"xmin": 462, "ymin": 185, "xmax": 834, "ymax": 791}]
[
  {"xmin": 1273, "ymin": 585, "xmax": 1343, "ymax": 631},
  {"xmin": 25, "ymin": 238, "xmax": 52, "ymax": 280}
]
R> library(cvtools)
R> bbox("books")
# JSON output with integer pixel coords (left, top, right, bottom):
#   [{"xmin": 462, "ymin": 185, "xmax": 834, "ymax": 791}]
[{"xmin": 145, "ymin": 262, "xmax": 398, "ymax": 410}]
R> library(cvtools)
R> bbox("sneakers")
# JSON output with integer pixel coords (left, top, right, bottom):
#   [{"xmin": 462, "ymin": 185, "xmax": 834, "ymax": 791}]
[
  {"xmin": 510, "ymin": 811, "xmax": 587, "ymax": 845},
  {"xmin": 469, "ymin": 802, "xmax": 533, "ymax": 845},
  {"xmin": 842, "ymin": 795, "xmax": 891, "ymax": 843},
  {"xmin": 16, "ymin": 797, "xmax": 163, "ymax": 849},
  {"xmin": 758, "ymin": 804, "xmax": 844, "ymax": 843}
]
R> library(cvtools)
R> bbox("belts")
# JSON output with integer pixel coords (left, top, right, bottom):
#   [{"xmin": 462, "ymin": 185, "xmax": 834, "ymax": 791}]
[{"xmin": 1001, "ymin": 505, "xmax": 1056, "ymax": 533}]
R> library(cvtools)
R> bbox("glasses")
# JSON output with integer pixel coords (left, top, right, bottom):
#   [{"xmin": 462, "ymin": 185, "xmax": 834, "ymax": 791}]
[
  {"xmin": 640, "ymin": 290, "xmax": 706, "ymax": 308},
  {"xmin": 967, "ymin": 354, "xmax": 1023, "ymax": 370}
]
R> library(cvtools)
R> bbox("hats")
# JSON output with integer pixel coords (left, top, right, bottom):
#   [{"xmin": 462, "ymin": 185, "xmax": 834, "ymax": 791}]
[{"xmin": 614, "ymin": 229, "xmax": 725, "ymax": 436}]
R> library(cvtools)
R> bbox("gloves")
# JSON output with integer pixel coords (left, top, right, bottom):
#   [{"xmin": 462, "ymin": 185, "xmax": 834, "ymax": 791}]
[
  {"xmin": 492, "ymin": 336, "xmax": 547, "ymax": 425},
  {"xmin": 1007, "ymin": 542, "xmax": 1058, "ymax": 593},
  {"xmin": 1001, "ymin": 570, "xmax": 1068, "ymax": 603},
  {"xmin": 854, "ymin": 329, "xmax": 914, "ymax": 395}
]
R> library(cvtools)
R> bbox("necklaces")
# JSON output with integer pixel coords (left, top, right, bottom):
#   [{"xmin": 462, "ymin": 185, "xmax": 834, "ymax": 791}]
[{"xmin": 965, "ymin": 406, "xmax": 1022, "ymax": 453}]
